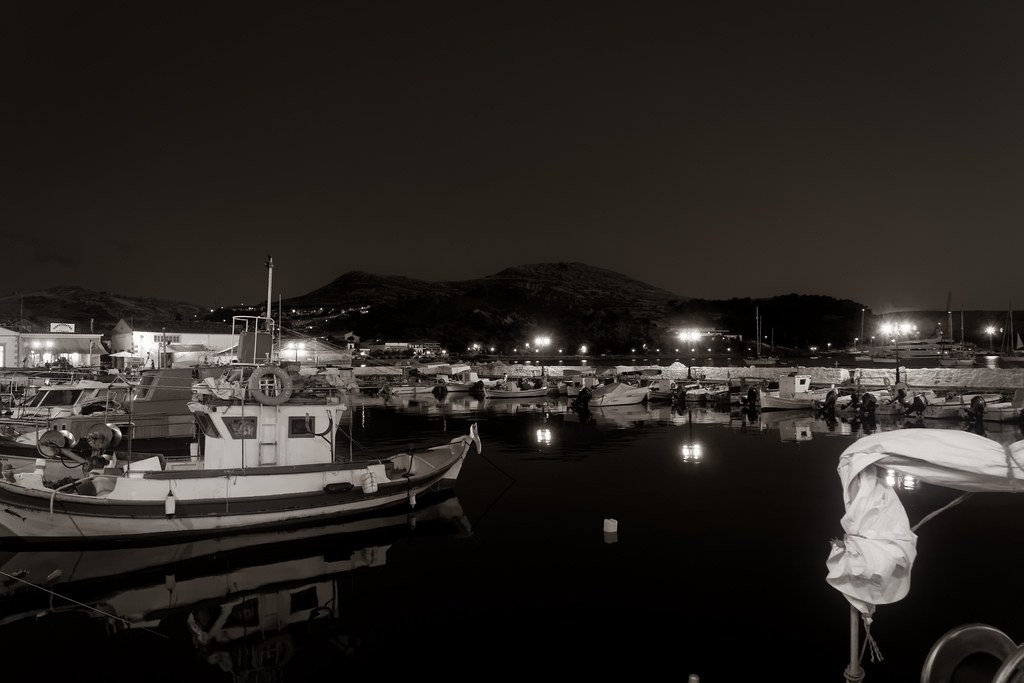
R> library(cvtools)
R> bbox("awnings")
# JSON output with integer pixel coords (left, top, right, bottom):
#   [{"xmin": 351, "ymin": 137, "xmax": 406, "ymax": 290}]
[
  {"xmin": 22, "ymin": 337, "xmax": 109, "ymax": 355},
  {"xmin": 160, "ymin": 345, "xmax": 211, "ymax": 354}
]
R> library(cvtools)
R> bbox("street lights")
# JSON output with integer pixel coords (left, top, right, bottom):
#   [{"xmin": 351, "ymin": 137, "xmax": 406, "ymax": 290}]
[
  {"xmin": 534, "ymin": 335, "xmax": 551, "ymax": 376},
  {"xmin": 853, "ymin": 309, "xmax": 875, "ymax": 354},
  {"xmin": 535, "ymin": 405, "xmax": 553, "ymax": 445},
  {"xmin": 882, "ymin": 320, "xmax": 911, "ymax": 384},
  {"xmin": 810, "ymin": 347, "xmax": 817, "ymax": 355},
  {"xmin": 987, "ymin": 326, "xmax": 996, "ymax": 353},
  {"xmin": 162, "ymin": 327, "xmax": 166, "ymax": 368},
  {"xmin": 680, "ymin": 410, "xmax": 704, "ymax": 464},
  {"xmin": 678, "ymin": 330, "xmax": 701, "ymax": 380},
  {"xmin": 827, "ymin": 343, "xmax": 832, "ymax": 351}
]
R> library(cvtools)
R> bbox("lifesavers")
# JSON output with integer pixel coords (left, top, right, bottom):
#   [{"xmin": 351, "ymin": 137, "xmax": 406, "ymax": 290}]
[
  {"xmin": 248, "ymin": 366, "xmax": 293, "ymax": 405},
  {"xmin": 252, "ymin": 634, "xmax": 295, "ymax": 674}
]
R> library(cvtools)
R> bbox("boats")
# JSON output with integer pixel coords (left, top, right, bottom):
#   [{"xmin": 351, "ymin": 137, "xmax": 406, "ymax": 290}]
[
  {"xmin": 0, "ymin": 254, "xmax": 481, "ymax": 538},
  {"xmin": 825, "ymin": 428, "xmax": 1023, "ymax": 683},
  {"xmin": 0, "ymin": 497, "xmax": 474, "ymax": 683},
  {"xmin": 811, "ymin": 390, "xmax": 1024, "ymax": 422},
  {"xmin": 383, "ymin": 362, "xmax": 839, "ymax": 410},
  {"xmin": 846, "ymin": 341, "xmax": 902, "ymax": 363}
]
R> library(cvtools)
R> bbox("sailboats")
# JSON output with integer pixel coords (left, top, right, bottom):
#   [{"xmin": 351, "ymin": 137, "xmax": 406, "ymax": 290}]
[
  {"xmin": 742, "ymin": 306, "xmax": 780, "ymax": 365},
  {"xmin": 936, "ymin": 306, "xmax": 977, "ymax": 367},
  {"xmin": 997, "ymin": 302, "xmax": 1024, "ymax": 362}
]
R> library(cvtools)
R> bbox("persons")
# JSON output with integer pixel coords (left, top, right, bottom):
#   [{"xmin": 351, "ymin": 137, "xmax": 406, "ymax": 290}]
[
  {"xmin": 203, "ymin": 356, "xmax": 223, "ymax": 364},
  {"xmin": 150, "ymin": 358, "xmax": 155, "ymax": 369},
  {"xmin": 45, "ymin": 360, "xmax": 50, "ymax": 368}
]
